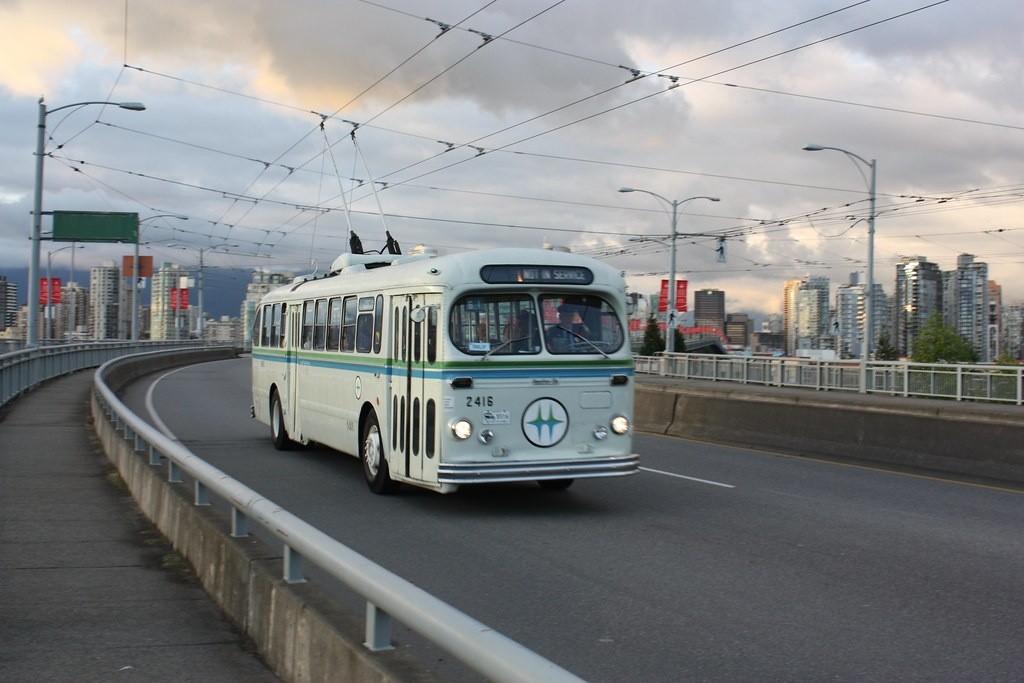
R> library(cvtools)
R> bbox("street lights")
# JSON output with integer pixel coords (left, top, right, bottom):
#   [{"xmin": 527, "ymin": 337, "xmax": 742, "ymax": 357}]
[
  {"xmin": 47, "ymin": 245, "xmax": 85, "ymax": 341},
  {"xmin": 166, "ymin": 242, "xmax": 239, "ymax": 341},
  {"xmin": 132, "ymin": 215, "xmax": 189, "ymax": 341},
  {"xmin": 27, "ymin": 101, "xmax": 146, "ymax": 348},
  {"xmin": 618, "ymin": 187, "xmax": 721, "ymax": 356},
  {"xmin": 802, "ymin": 143, "xmax": 878, "ymax": 394}
]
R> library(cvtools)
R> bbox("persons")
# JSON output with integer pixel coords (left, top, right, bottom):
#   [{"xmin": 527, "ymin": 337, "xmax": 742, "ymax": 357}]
[
  {"xmin": 474, "ymin": 304, "xmax": 592, "ymax": 352},
  {"xmin": 304, "ymin": 327, "xmax": 312, "ymax": 349}
]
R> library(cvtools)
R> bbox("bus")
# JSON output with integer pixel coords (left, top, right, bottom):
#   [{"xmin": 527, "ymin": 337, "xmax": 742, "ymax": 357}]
[{"xmin": 248, "ymin": 244, "xmax": 641, "ymax": 497}]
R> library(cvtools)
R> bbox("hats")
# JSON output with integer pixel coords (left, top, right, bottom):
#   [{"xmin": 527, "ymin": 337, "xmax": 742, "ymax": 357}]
[{"xmin": 557, "ymin": 304, "xmax": 580, "ymax": 312}]
